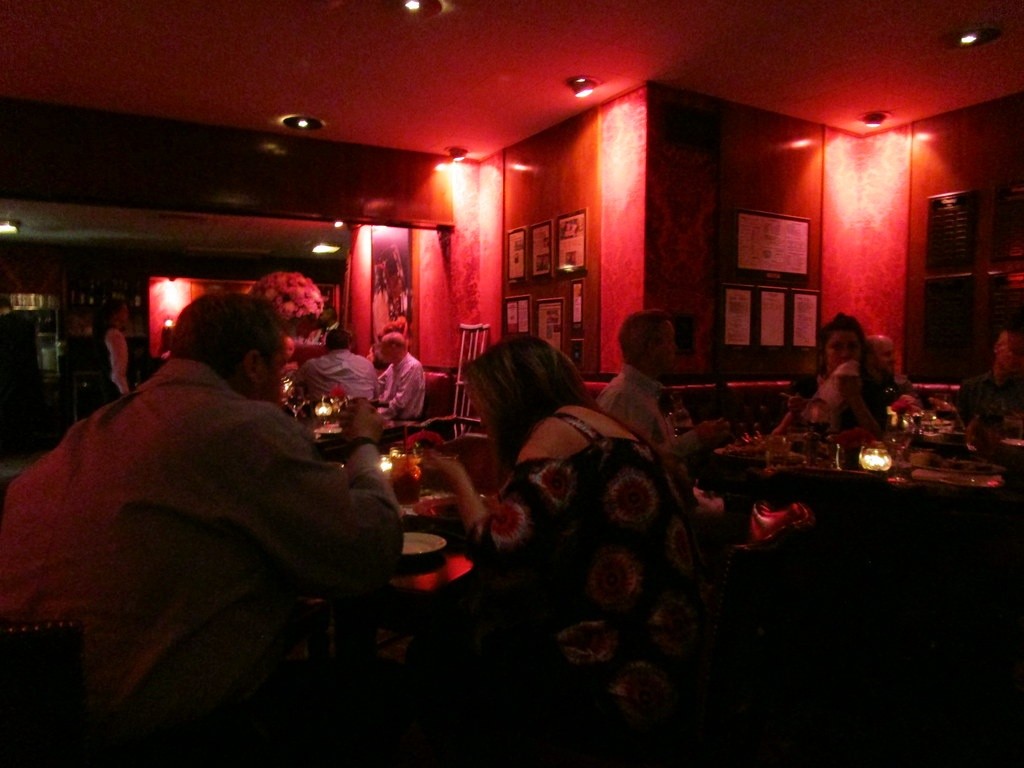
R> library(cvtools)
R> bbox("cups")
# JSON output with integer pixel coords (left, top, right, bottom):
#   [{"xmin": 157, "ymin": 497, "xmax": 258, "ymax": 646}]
[{"xmin": 390, "ymin": 447, "xmax": 423, "ymax": 506}]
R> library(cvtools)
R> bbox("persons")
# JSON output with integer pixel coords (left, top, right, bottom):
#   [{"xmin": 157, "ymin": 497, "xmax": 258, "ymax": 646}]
[
  {"xmin": 956, "ymin": 323, "xmax": 1024, "ymax": 440},
  {"xmin": 404, "ymin": 334, "xmax": 706, "ymax": 768},
  {"xmin": 599, "ymin": 311, "xmax": 728, "ymax": 505},
  {"xmin": 0, "ymin": 291, "xmax": 403, "ymax": 767},
  {"xmin": 384, "ymin": 331, "xmax": 425, "ymax": 414},
  {"xmin": 773, "ymin": 312, "xmax": 920, "ymax": 436},
  {"xmin": 91, "ymin": 298, "xmax": 136, "ymax": 406},
  {"xmin": 292, "ymin": 329, "xmax": 378, "ymax": 403}
]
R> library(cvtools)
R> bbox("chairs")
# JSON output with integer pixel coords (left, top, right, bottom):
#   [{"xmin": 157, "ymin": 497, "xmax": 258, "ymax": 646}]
[{"xmin": 0, "ymin": 366, "xmax": 961, "ymax": 768}]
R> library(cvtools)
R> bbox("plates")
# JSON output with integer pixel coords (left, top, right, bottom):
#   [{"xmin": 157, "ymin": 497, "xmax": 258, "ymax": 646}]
[
  {"xmin": 313, "ymin": 424, "xmax": 342, "ymax": 434},
  {"xmin": 913, "ymin": 461, "xmax": 1009, "ymax": 474},
  {"xmin": 715, "ymin": 448, "xmax": 806, "ymax": 463},
  {"xmin": 402, "ymin": 533, "xmax": 447, "ymax": 557}
]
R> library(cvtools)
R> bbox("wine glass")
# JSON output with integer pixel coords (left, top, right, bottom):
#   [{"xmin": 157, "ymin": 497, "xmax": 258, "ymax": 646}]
[
  {"xmin": 287, "ymin": 389, "xmax": 305, "ymax": 418},
  {"xmin": 886, "ymin": 432, "xmax": 913, "ymax": 481}
]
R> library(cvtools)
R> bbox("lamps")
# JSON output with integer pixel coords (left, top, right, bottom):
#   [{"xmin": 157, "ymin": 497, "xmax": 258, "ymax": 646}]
[
  {"xmin": 565, "ymin": 75, "xmax": 602, "ymax": 98},
  {"xmin": 448, "ymin": 147, "xmax": 469, "ymax": 161},
  {"xmin": 857, "ymin": 112, "xmax": 892, "ymax": 126}
]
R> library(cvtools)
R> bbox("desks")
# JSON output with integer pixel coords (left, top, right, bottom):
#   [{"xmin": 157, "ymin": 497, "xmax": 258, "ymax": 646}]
[
  {"xmin": 713, "ymin": 433, "xmax": 1008, "ymax": 512},
  {"xmin": 297, "ymin": 413, "xmax": 419, "ymax": 459}
]
